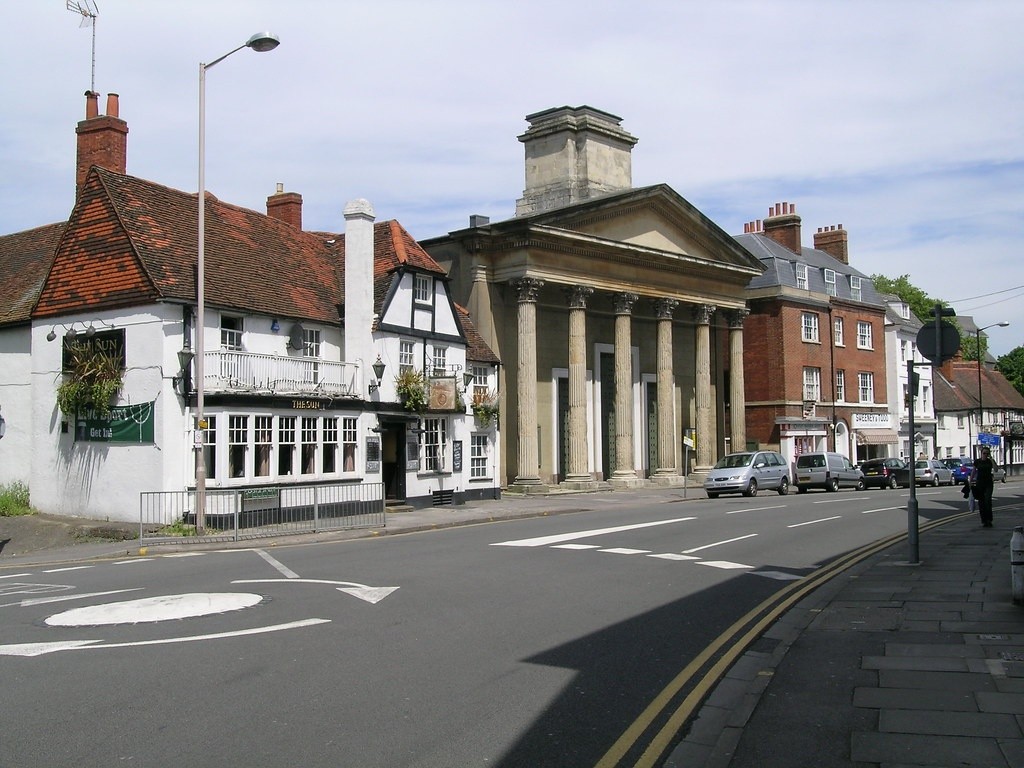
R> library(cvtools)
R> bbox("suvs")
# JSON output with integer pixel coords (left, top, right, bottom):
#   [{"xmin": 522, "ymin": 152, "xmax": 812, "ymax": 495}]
[{"xmin": 703, "ymin": 448, "xmax": 790, "ymax": 498}]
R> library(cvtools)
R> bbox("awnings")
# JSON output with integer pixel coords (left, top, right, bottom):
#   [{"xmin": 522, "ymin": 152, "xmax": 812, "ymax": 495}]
[{"xmin": 856, "ymin": 428, "xmax": 899, "ymax": 446}]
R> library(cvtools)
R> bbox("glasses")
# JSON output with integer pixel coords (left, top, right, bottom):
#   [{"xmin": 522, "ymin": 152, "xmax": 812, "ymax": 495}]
[{"xmin": 983, "ymin": 453, "xmax": 990, "ymax": 455}]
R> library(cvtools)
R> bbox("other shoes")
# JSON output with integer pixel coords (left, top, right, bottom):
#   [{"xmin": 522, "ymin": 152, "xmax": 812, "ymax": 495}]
[{"xmin": 983, "ymin": 521, "xmax": 993, "ymax": 528}]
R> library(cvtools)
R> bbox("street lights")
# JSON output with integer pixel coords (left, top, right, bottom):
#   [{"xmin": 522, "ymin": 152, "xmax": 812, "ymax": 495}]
[
  {"xmin": 191, "ymin": 31, "xmax": 281, "ymax": 535},
  {"xmin": 976, "ymin": 320, "xmax": 1013, "ymax": 431}
]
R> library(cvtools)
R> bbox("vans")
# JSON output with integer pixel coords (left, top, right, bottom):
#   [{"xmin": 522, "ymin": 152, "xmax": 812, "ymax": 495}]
[{"xmin": 793, "ymin": 452, "xmax": 865, "ymax": 494}]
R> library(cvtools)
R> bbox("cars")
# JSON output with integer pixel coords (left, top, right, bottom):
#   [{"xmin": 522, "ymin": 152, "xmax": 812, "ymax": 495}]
[
  {"xmin": 939, "ymin": 457, "xmax": 978, "ymax": 486},
  {"xmin": 914, "ymin": 459, "xmax": 956, "ymax": 487},
  {"xmin": 859, "ymin": 456, "xmax": 910, "ymax": 490},
  {"xmin": 991, "ymin": 468, "xmax": 1007, "ymax": 483}
]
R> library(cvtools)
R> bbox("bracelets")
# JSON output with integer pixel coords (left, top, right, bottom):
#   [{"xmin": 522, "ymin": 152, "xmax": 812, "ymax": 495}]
[{"xmin": 989, "ymin": 456, "xmax": 992, "ymax": 460}]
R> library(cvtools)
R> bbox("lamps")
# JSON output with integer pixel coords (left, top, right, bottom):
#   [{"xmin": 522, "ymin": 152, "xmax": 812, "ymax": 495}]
[
  {"xmin": 47, "ymin": 317, "xmax": 115, "ymax": 341},
  {"xmin": 172, "ymin": 338, "xmax": 195, "ymax": 389},
  {"xmin": 368, "ymin": 354, "xmax": 386, "ymax": 395}
]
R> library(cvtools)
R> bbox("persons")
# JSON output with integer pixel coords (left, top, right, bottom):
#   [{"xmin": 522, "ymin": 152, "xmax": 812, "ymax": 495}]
[
  {"xmin": 917, "ymin": 452, "xmax": 928, "ymax": 467},
  {"xmin": 969, "ymin": 447, "xmax": 998, "ymax": 528}
]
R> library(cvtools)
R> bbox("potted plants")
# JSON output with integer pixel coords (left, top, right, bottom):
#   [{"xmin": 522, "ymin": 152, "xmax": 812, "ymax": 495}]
[
  {"xmin": 469, "ymin": 393, "xmax": 497, "ymax": 426},
  {"xmin": 396, "ymin": 367, "xmax": 433, "ymax": 411}
]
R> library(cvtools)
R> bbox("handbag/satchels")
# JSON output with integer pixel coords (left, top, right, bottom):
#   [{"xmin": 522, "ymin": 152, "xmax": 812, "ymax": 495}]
[{"xmin": 969, "ymin": 489, "xmax": 975, "ymax": 512}]
